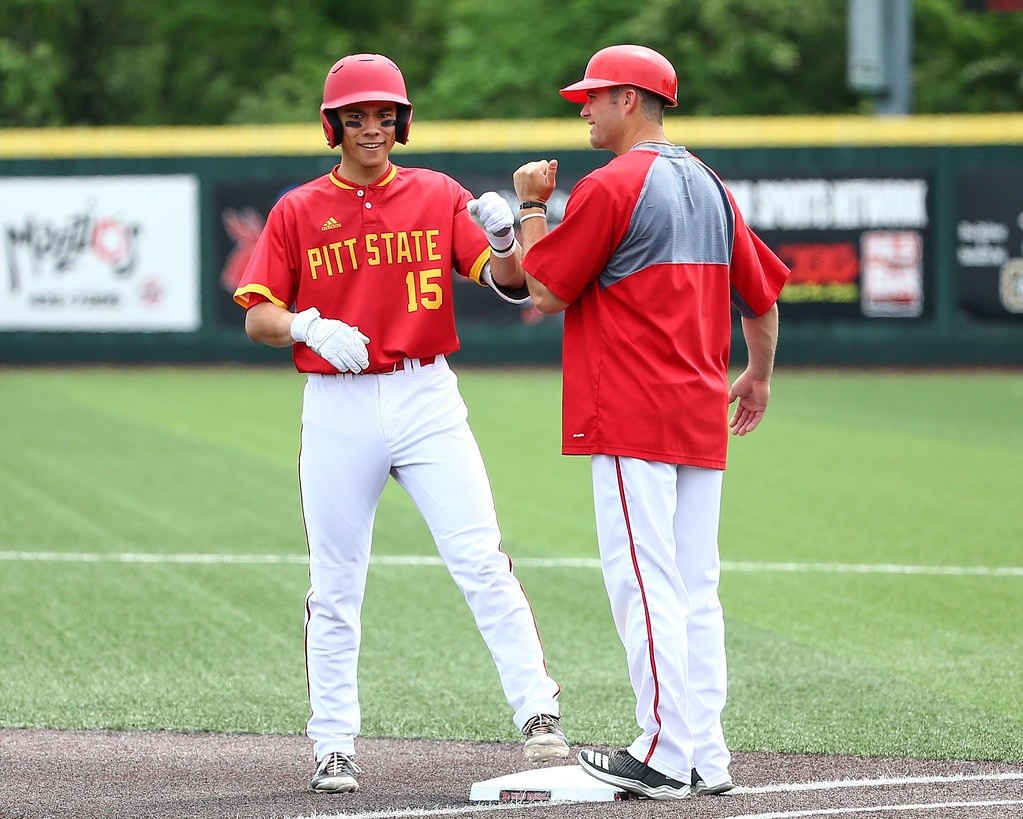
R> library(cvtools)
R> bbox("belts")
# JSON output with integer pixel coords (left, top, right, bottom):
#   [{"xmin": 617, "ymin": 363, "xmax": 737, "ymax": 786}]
[{"xmin": 363, "ymin": 356, "xmax": 435, "ymax": 374}]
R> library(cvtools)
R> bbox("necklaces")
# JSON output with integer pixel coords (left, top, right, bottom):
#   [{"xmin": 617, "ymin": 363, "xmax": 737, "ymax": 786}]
[{"xmin": 629, "ymin": 140, "xmax": 675, "ymax": 150}]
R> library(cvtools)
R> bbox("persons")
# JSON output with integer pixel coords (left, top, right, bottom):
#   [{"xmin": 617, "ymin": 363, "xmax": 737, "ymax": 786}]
[
  {"xmin": 233, "ymin": 54, "xmax": 569, "ymax": 793},
  {"xmin": 513, "ymin": 45, "xmax": 792, "ymax": 800}
]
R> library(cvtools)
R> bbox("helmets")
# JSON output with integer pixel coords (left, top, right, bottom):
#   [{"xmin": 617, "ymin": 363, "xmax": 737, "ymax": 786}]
[
  {"xmin": 320, "ymin": 53, "xmax": 413, "ymax": 145},
  {"xmin": 559, "ymin": 44, "xmax": 678, "ymax": 107}
]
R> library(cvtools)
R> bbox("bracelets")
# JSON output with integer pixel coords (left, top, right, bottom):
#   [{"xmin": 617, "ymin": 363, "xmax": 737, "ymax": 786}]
[
  {"xmin": 520, "ymin": 213, "xmax": 546, "ymax": 224},
  {"xmin": 520, "ymin": 201, "xmax": 547, "ymax": 214}
]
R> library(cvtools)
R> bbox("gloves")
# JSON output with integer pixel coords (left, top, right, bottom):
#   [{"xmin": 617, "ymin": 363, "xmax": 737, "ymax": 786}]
[
  {"xmin": 291, "ymin": 307, "xmax": 372, "ymax": 374},
  {"xmin": 466, "ymin": 191, "xmax": 514, "ymax": 236}
]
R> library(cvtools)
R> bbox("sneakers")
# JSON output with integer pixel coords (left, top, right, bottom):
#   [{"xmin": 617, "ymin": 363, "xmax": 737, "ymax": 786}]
[
  {"xmin": 691, "ymin": 766, "xmax": 734, "ymax": 794},
  {"xmin": 578, "ymin": 748, "xmax": 691, "ymax": 800},
  {"xmin": 522, "ymin": 715, "xmax": 569, "ymax": 760},
  {"xmin": 311, "ymin": 752, "xmax": 362, "ymax": 794}
]
